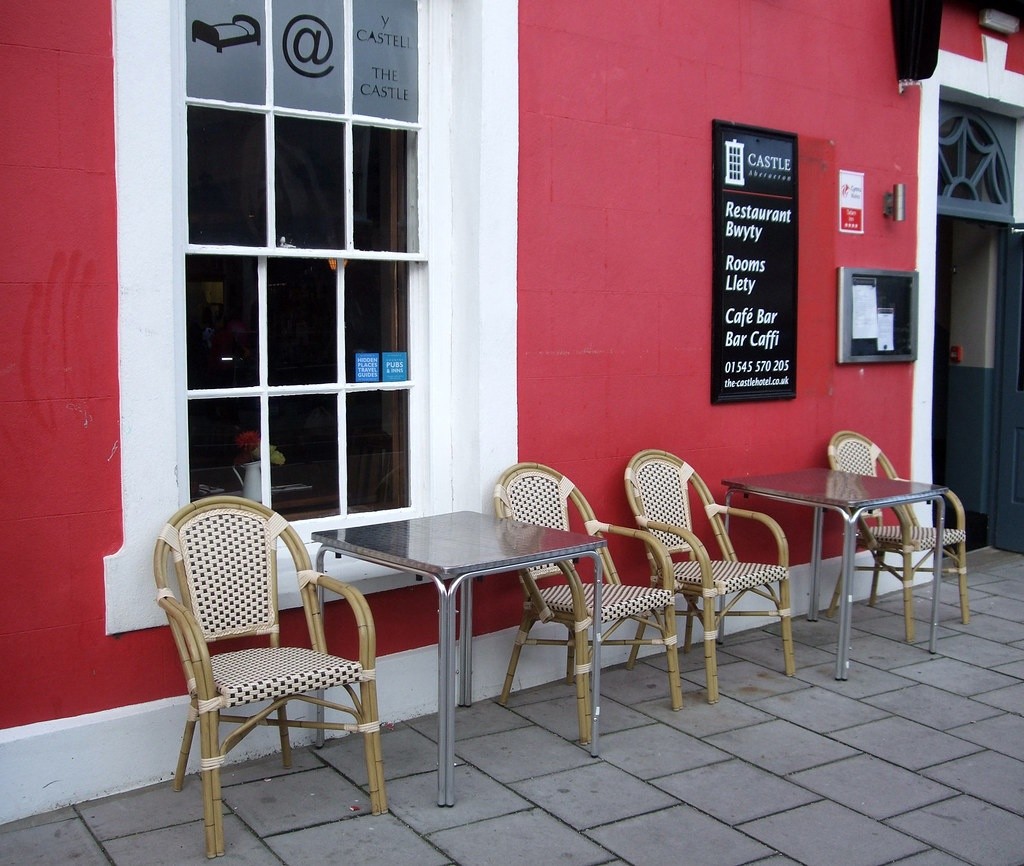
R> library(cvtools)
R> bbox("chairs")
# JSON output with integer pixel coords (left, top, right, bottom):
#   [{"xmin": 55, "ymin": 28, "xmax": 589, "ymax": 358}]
[
  {"xmin": 624, "ymin": 447, "xmax": 793, "ymax": 705},
  {"xmin": 494, "ymin": 462, "xmax": 683, "ymax": 744},
  {"xmin": 155, "ymin": 495, "xmax": 391, "ymax": 859},
  {"xmin": 826, "ymin": 430, "xmax": 969, "ymax": 643},
  {"xmin": 715, "ymin": 467, "xmax": 948, "ymax": 679},
  {"xmin": 233, "ymin": 460, "xmax": 262, "ymax": 503}
]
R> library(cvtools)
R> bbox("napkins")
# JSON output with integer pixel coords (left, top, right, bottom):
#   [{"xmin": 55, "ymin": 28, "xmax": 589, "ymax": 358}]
[
  {"xmin": 198, "ymin": 485, "xmax": 224, "ymax": 494},
  {"xmin": 268, "ymin": 483, "xmax": 312, "ymax": 492}
]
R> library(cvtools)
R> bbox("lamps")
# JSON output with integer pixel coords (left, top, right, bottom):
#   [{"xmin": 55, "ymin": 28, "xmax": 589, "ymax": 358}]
[
  {"xmin": 979, "ymin": 8, "xmax": 1021, "ymax": 34},
  {"xmin": 883, "ymin": 183, "xmax": 905, "ymax": 221}
]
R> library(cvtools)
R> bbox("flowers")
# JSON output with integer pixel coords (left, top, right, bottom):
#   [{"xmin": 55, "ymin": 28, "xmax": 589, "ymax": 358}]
[{"xmin": 237, "ymin": 433, "xmax": 286, "ymax": 466}]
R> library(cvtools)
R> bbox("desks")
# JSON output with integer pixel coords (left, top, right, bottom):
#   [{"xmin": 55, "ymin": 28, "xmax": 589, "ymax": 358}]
[{"xmin": 309, "ymin": 511, "xmax": 607, "ymax": 807}]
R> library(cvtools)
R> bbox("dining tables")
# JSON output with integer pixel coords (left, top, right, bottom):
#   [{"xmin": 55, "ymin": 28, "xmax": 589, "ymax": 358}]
[{"xmin": 191, "ymin": 477, "xmax": 368, "ymax": 520}]
